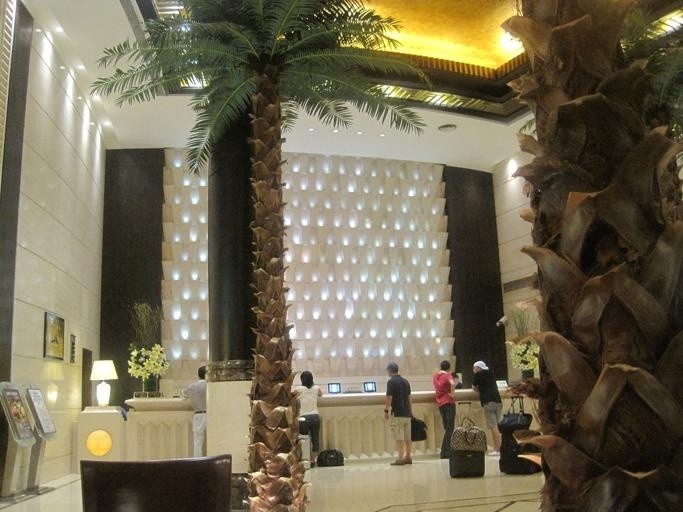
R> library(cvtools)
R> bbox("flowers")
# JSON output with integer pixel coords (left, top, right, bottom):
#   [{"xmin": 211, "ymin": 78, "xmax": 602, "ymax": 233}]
[
  {"xmin": 505, "ymin": 343, "xmax": 540, "ymax": 371},
  {"xmin": 126, "ymin": 344, "xmax": 169, "ymax": 381}
]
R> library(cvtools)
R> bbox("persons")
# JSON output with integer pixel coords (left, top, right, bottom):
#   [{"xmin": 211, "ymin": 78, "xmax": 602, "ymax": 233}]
[
  {"xmin": 432, "ymin": 360, "xmax": 456, "ymax": 459},
  {"xmin": 182, "ymin": 366, "xmax": 207, "ymax": 458},
  {"xmin": 471, "ymin": 360, "xmax": 502, "ymax": 455},
  {"xmin": 384, "ymin": 363, "xmax": 412, "ymax": 465},
  {"xmin": 291, "ymin": 371, "xmax": 322, "ymax": 468},
  {"xmin": 451, "ymin": 373, "xmax": 462, "ymax": 389}
]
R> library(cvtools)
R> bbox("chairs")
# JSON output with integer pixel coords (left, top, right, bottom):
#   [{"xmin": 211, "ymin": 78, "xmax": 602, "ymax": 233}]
[{"xmin": 77, "ymin": 450, "xmax": 232, "ymax": 512}]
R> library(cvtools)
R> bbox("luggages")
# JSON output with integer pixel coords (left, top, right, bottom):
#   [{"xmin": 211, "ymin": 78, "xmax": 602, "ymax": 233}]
[{"xmin": 499, "ymin": 431, "xmax": 543, "ymax": 475}]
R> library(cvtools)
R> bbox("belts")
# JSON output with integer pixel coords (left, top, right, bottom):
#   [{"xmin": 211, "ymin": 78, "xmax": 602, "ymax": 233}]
[{"xmin": 195, "ymin": 410, "xmax": 207, "ymax": 414}]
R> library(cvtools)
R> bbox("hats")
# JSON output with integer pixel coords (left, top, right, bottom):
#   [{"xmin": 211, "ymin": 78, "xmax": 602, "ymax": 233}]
[
  {"xmin": 386, "ymin": 362, "xmax": 399, "ymax": 371},
  {"xmin": 473, "ymin": 360, "xmax": 489, "ymax": 370}
]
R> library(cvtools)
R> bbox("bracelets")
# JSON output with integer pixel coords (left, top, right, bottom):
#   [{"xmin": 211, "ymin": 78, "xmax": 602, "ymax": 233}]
[{"xmin": 384, "ymin": 410, "xmax": 388, "ymax": 413}]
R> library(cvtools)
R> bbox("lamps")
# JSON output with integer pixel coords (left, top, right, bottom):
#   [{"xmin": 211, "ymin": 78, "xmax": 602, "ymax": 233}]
[
  {"xmin": 88, "ymin": 359, "xmax": 120, "ymax": 408},
  {"xmin": 38, "ymin": 360, "xmax": 66, "ymax": 404}
]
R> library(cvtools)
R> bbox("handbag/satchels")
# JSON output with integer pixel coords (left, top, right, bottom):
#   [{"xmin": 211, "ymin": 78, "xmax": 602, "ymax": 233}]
[
  {"xmin": 317, "ymin": 449, "xmax": 345, "ymax": 467},
  {"xmin": 497, "ymin": 413, "xmax": 533, "ymax": 434},
  {"xmin": 449, "ymin": 449, "xmax": 485, "ymax": 478},
  {"xmin": 448, "ymin": 415, "xmax": 487, "ymax": 452},
  {"xmin": 411, "ymin": 415, "xmax": 428, "ymax": 442}
]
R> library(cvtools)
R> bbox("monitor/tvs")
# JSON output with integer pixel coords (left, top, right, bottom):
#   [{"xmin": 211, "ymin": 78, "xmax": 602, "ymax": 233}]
[
  {"xmin": 328, "ymin": 384, "xmax": 341, "ymax": 394},
  {"xmin": 364, "ymin": 381, "xmax": 376, "ymax": 392}
]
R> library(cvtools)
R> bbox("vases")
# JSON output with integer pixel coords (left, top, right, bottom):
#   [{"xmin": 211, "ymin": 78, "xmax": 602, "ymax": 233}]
[
  {"xmin": 142, "ymin": 375, "xmax": 159, "ymax": 392},
  {"xmin": 520, "ymin": 368, "xmax": 534, "ymax": 379}
]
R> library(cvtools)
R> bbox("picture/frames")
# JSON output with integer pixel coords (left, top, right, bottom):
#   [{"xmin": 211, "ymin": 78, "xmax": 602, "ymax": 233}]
[{"xmin": 42, "ymin": 311, "xmax": 65, "ymax": 360}]
